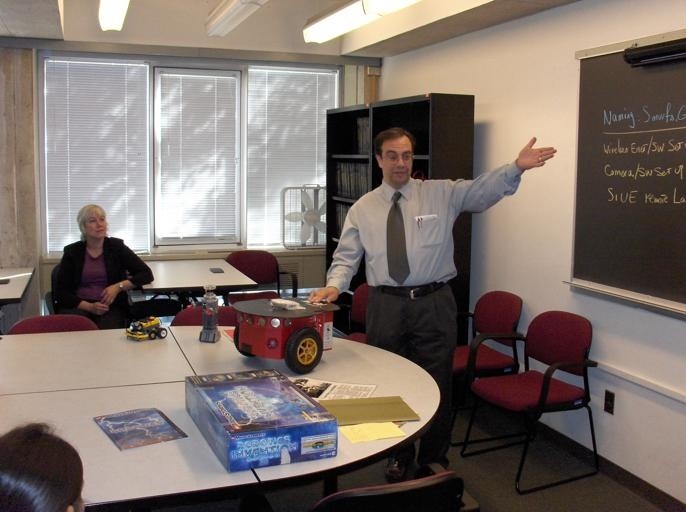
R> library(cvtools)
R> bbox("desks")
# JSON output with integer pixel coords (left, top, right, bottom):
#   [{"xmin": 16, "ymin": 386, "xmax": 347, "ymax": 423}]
[
  {"xmin": 0, "ymin": 326, "xmax": 441, "ymax": 512},
  {"xmin": 140, "ymin": 259, "xmax": 259, "ymax": 311},
  {"xmin": 0, "ymin": 267, "xmax": 41, "ymax": 334}
]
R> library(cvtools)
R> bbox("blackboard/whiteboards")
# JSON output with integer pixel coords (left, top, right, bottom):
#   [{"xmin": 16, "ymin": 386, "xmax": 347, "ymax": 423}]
[{"xmin": 561, "ymin": 27, "xmax": 686, "ymax": 316}]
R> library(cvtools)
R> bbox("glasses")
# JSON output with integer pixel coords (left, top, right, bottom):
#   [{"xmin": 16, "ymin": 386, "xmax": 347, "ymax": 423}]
[{"xmin": 381, "ymin": 153, "xmax": 415, "ymax": 163}]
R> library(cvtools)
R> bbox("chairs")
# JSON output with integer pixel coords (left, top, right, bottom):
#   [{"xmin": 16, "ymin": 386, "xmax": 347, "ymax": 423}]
[
  {"xmin": 313, "ymin": 459, "xmax": 480, "ymax": 512},
  {"xmin": 341, "ymin": 282, "xmax": 370, "ymax": 341},
  {"xmin": 5, "ymin": 314, "xmax": 98, "ymax": 334},
  {"xmin": 221, "ymin": 251, "xmax": 299, "ymax": 308},
  {"xmin": 171, "ymin": 304, "xmax": 236, "ymax": 327},
  {"xmin": 50, "ymin": 262, "xmax": 60, "ymax": 315},
  {"xmin": 451, "ymin": 290, "xmax": 524, "ymax": 448},
  {"xmin": 461, "ymin": 312, "xmax": 599, "ymax": 496}
]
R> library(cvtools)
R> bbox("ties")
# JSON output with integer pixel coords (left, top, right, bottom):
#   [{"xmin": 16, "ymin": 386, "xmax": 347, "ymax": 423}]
[{"xmin": 386, "ymin": 192, "xmax": 411, "ymax": 284}]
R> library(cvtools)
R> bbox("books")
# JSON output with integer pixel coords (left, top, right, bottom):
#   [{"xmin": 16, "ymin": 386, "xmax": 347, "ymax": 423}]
[
  {"xmin": 336, "ymin": 162, "xmax": 368, "ymax": 200},
  {"xmin": 357, "ymin": 118, "xmax": 371, "ymax": 154},
  {"xmin": 336, "ymin": 203, "xmax": 352, "ymax": 239}
]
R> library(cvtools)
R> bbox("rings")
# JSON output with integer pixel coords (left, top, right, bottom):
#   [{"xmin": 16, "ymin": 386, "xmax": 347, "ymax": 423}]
[{"xmin": 539, "ymin": 157, "xmax": 542, "ymax": 162}]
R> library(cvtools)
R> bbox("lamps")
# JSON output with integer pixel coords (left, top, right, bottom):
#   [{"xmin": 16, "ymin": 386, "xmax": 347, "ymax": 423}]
[
  {"xmin": 300, "ymin": 1, "xmax": 423, "ymax": 45},
  {"xmin": 98, "ymin": 0, "xmax": 130, "ymax": 33},
  {"xmin": 205, "ymin": 0, "xmax": 269, "ymax": 38}
]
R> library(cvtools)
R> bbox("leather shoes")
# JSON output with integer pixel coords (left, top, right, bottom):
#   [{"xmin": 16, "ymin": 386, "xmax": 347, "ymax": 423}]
[
  {"xmin": 417, "ymin": 459, "xmax": 448, "ymax": 476},
  {"xmin": 386, "ymin": 457, "xmax": 407, "ymax": 481}
]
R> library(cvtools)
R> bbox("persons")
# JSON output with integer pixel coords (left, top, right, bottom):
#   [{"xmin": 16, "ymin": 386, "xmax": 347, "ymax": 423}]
[
  {"xmin": 51, "ymin": 205, "xmax": 154, "ymax": 330},
  {"xmin": 0, "ymin": 423, "xmax": 84, "ymax": 512},
  {"xmin": 308, "ymin": 128, "xmax": 557, "ymax": 485}
]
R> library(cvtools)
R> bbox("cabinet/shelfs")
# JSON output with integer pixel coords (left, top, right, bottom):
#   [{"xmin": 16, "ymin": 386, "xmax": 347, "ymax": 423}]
[{"xmin": 326, "ymin": 92, "xmax": 474, "ymax": 346}]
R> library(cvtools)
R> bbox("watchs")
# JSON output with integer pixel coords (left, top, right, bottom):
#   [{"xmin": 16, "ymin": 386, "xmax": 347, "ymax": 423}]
[{"xmin": 118, "ymin": 282, "xmax": 123, "ymax": 291}]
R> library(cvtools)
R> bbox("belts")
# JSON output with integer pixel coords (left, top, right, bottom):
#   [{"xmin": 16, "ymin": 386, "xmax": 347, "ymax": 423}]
[{"xmin": 379, "ymin": 281, "xmax": 445, "ymax": 300}]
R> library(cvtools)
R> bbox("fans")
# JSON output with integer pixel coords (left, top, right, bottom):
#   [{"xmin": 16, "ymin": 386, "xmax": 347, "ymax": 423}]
[{"xmin": 279, "ymin": 183, "xmax": 326, "ymax": 250}]
